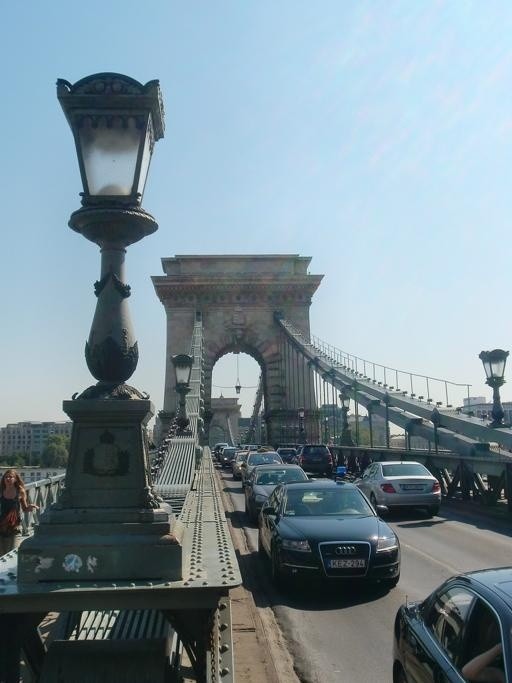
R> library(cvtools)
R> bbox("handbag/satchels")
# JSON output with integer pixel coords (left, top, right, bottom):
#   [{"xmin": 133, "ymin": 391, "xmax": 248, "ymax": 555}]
[{"xmin": 0, "ymin": 507, "xmax": 18, "ymax": 535}]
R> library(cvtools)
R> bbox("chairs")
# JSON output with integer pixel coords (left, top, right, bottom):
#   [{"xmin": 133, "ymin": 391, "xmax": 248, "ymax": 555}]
[{"xmin": 295, "ymin": 503, "xmax": 312, "ymax": 515}]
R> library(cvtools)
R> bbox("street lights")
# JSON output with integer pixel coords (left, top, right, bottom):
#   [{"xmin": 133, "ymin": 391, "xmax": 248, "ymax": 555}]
[
  {"xmin": 165, "ymin": 350, "xmax": 197, "ymax": 440},
  {"xmin": 19, "ymin": 69, "xmax": 178, "ymax": 585},
  {"xmin": 332, "ymin": 386, "xmax": 360, "ymax": 449},
  {"xmin": 292, "ymin": 403, "xmax": 310, "ymax": 444},
  {"xmin": 476, "ymin": 343, "xmax": 512, "ymax": 431}
]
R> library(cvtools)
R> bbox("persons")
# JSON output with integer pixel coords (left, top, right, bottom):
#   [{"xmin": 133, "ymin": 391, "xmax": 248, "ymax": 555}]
[
  {"xmin": 461, "ymin": 642, "xmax": 506, "ymax": 682},
  {"xmin": 1, "ymin": 469, "xmax": 41, "ymax": 555}
]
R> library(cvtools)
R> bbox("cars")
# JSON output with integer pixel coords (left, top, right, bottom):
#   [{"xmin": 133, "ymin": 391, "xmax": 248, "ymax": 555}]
[
  {"xmin": 209, "ymin": 440, "xmax": 444, "ymax": 594},
  {"xmin": 387, "ymin": 564, "xmax": 512, "ymax": 683}
]
